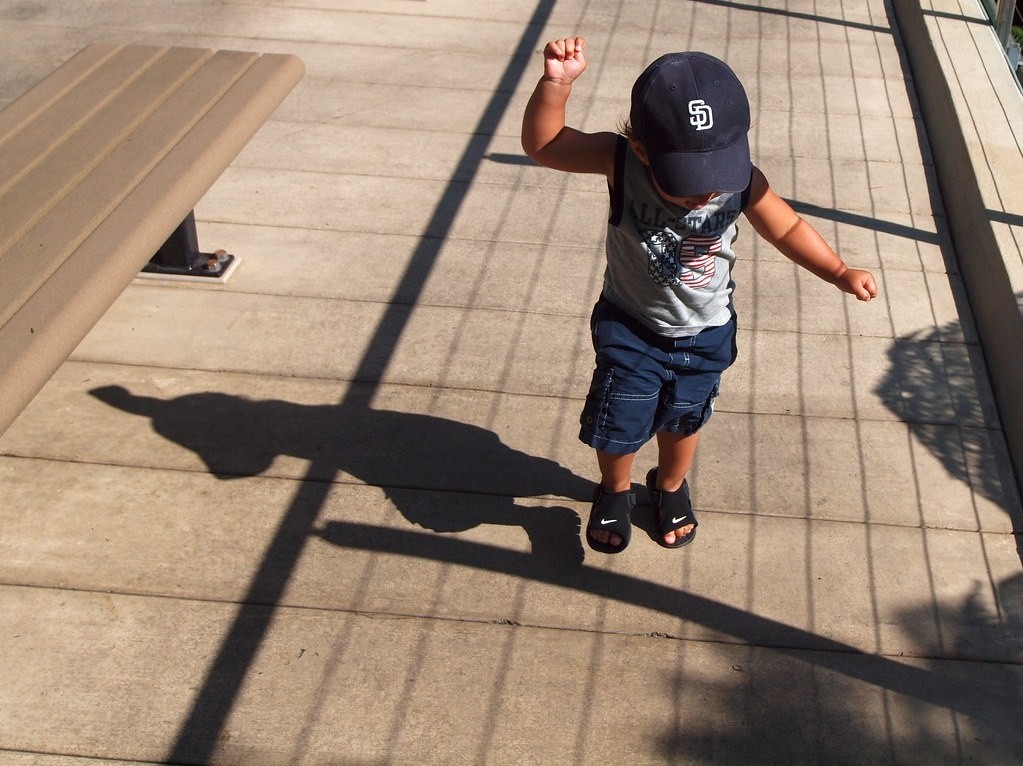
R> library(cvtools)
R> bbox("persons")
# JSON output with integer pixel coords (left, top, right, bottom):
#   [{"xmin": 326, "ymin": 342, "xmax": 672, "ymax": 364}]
[{"xmin": 520, "ymin": 37, "xmax": 879, "ymax": 554}]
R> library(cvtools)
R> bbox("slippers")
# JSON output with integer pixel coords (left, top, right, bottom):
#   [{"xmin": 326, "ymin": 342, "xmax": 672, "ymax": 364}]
[
  {"xmin": 647, "ymin": 466, "xmax": 698, "ymax": 549},
  {"xmin": 586, "ymin": 482, "xmax": 636, "ymax": 554}
]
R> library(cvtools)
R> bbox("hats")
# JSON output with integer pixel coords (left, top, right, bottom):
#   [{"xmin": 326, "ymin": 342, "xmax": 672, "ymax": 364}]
[{"xmin": 630, "ymin": 51, "xmax": 752, "ymax": 198}]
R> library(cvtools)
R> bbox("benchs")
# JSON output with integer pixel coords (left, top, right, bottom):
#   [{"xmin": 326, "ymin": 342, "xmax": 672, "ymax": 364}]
[{"xmin": 0, "ymin": 44, "xmax": 303, "ymax": 434}]
